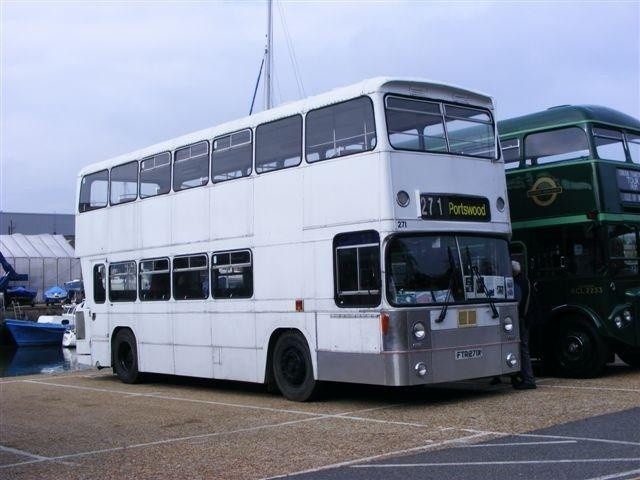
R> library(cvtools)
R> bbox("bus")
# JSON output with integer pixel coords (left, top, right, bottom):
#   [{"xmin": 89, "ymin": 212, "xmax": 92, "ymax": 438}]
[
  {"xmin": 63, "ymin": 70, "xmax": 532, "ymax": 406},
  {"xmin": 385, "ymin": 100, "xmax": 640, "ymax": 383}
]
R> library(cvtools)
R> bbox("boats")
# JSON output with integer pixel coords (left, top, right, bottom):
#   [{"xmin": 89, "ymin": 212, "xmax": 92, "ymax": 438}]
[
  {"xmin": 3, "ymin": 278, "xmax": 85, "ymax": 348},
  {"xmin": 2, "ymin": 345, "xmax": 91, "ymax": 376}
]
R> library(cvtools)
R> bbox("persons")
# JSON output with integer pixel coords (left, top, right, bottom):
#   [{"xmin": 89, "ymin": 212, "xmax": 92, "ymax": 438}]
[{"xmin": 511, "ymin": 259, "xmax": 537, "ymax": 391}]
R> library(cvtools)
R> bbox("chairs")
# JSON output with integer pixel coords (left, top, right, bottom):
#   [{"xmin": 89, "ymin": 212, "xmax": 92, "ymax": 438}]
[{"xmin": 391, "ymin": 261, "xmax": 410, "ymax": 288}]
